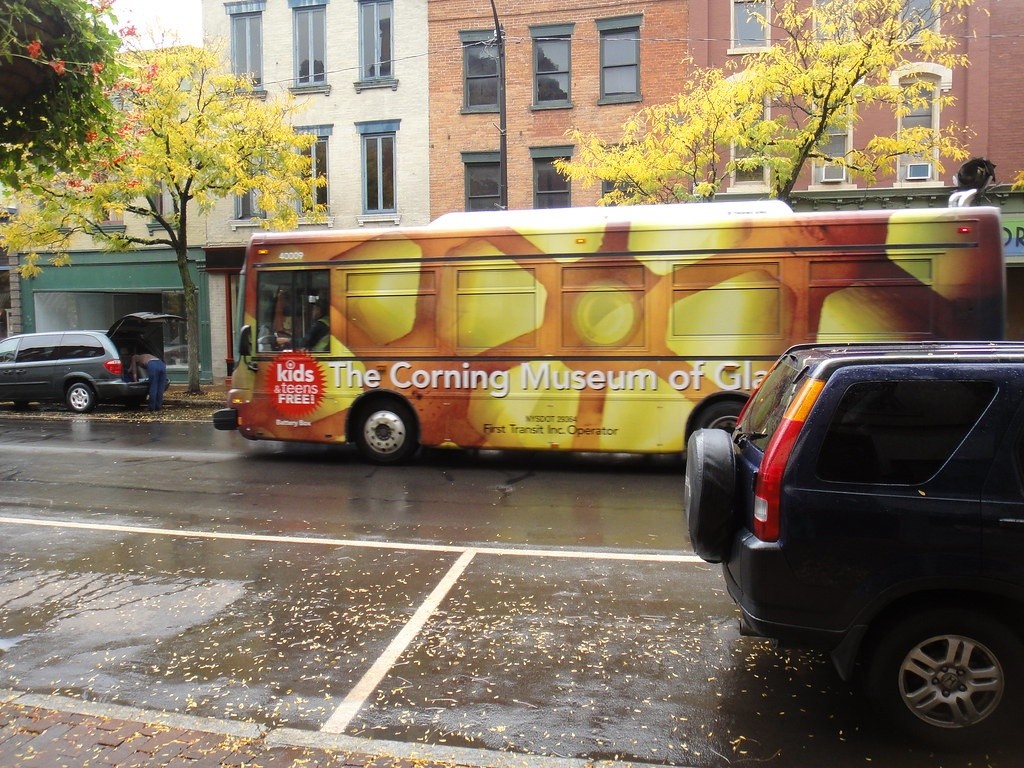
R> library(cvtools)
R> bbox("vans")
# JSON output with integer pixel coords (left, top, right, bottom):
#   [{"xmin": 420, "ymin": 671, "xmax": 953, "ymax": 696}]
[{"xmin": 0, "ymin": 311, "xmax": 187, "ymax": 414}]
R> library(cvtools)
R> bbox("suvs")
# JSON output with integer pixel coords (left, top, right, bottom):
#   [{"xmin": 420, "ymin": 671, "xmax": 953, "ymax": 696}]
[{"xmin": 683, "ymin": 340, "xmax": 1024, "ymax": 742}]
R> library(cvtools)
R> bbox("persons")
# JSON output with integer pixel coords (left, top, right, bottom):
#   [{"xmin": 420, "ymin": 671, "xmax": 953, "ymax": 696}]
[
  {"xmin": 127, "ymin": 353, "xmax": 166, "ymax": 412},
  {"xmin": 275, "ymin": 299, "xmax": 330, "ymax": 351}
]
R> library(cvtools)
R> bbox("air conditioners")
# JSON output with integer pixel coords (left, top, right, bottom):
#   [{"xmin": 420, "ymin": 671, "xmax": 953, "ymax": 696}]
[
  {"xmin": 905, "ymin": 163, "xmax": 930, "ymax": 179},
  {"xmin": 820, "ymin": 164, "xmax": 846, "ymax": 182}
]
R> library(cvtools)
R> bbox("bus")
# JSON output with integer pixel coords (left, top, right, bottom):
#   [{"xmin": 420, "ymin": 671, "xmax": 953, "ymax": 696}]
[{"xmin": 214, "ymin": 199, "xmax": 1005, "ymax": 465}]
